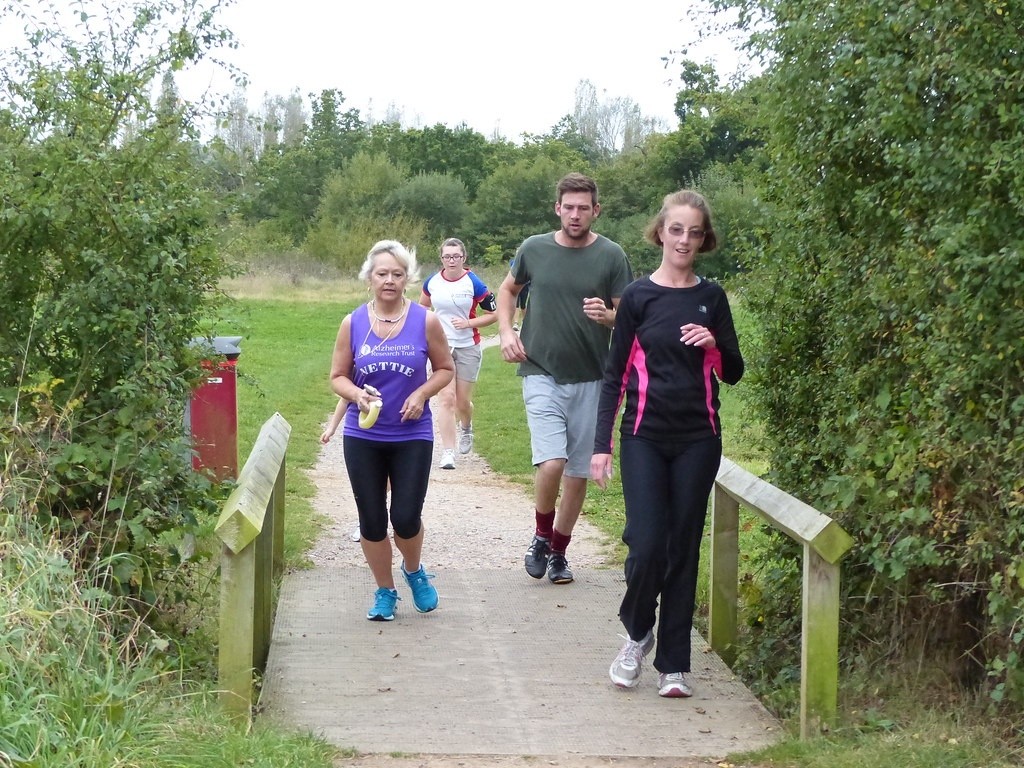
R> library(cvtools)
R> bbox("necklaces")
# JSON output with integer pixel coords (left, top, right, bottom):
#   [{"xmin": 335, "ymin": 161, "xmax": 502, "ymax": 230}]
[
  {"xmin": 356, "ymin": 296, "xmax": 405, "ymax": 360},
  {"xmin": 371, "ymin": 296, "xmax": 407, "ymax": 323}
]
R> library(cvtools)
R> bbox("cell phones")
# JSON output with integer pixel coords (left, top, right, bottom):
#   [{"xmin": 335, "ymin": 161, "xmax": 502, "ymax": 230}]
[{"xmin": 364, "ymin": 384, "xmax": 377, "ymax": 397}]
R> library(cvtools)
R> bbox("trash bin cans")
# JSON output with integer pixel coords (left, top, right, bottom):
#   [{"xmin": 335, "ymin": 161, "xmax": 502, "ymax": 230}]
[{"xmin": 180, "ymin": 336, "xmax": 243, "ymax": 488}]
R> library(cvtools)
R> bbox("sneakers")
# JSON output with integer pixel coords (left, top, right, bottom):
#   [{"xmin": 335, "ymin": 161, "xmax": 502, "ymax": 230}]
[
  {"xmin": 401, "ymin": 558, "xmax": 439, "ymax": 613},
  {"xmin": 609, "ymin": 628, "xmax": 655, "ymax": 688},
  {"xmin": 657, "ymin": 672, "xmax": 692, "ymax": 697},
  {"xmin": 548, "ymin": 553, "xmax": 573, "ymax": 583},
  {"xmin": 366, "ymin": 586, "xmax": 401, "ymax": 621},
  {"xmin": 524, "ymin": 534, "xmax": 551, "ymax": 579},
  {"xmin": 352, "ymin": 523, "xmax": 361, "ymax": 542},
  {"xmin": 459, "ymin": 419, "xmax": 473, "ymax": 454},
  {"xmin": 513, "ymin": 325, "xmax": 519, "ymax": 331},
  {"xmin": 439, "ymin": 449, "xmax": 456, "ymax": 469}
]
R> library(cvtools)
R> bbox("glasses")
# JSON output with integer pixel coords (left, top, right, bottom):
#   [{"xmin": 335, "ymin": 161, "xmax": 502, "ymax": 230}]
[
  {"xmin": 442, "ymin": 255, "xmax": 464, "ymax": 261},
  {"xmin": 663, "ymin": 224, "xmax": 704, "ymax": 239}
]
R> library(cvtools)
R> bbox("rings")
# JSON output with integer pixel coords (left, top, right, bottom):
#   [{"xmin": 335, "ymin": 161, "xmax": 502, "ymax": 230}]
[
  {"xmin": 701, "ymin": 333, "xmax": 705, "ymax": 337},
  {"xmin": 407, "ymin": 409, "xmax": 414, "ymax": 413}
]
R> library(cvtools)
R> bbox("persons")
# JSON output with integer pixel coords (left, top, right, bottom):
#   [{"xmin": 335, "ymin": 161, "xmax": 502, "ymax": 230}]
[
  {"xmin": 329, "ymin": 240, "xmax": 455, "ymax": 621},
  {"xmin": 504, "ymin": 245, "xmax": 532, "ymax": 331},
  {"xmin": 418, "ymin": 238, "xmax": 498, "ymax": 470},
  {"xmin": 321, "ymin": 393, "xmax": 393, "ymax": 497},
  {"xmin": 591, "ymin": 191, "xmax": 744, "ymax": 698},
  {"xmin": 494, "ymin": 172, "xmax": 634, "ymax": 584}
]
